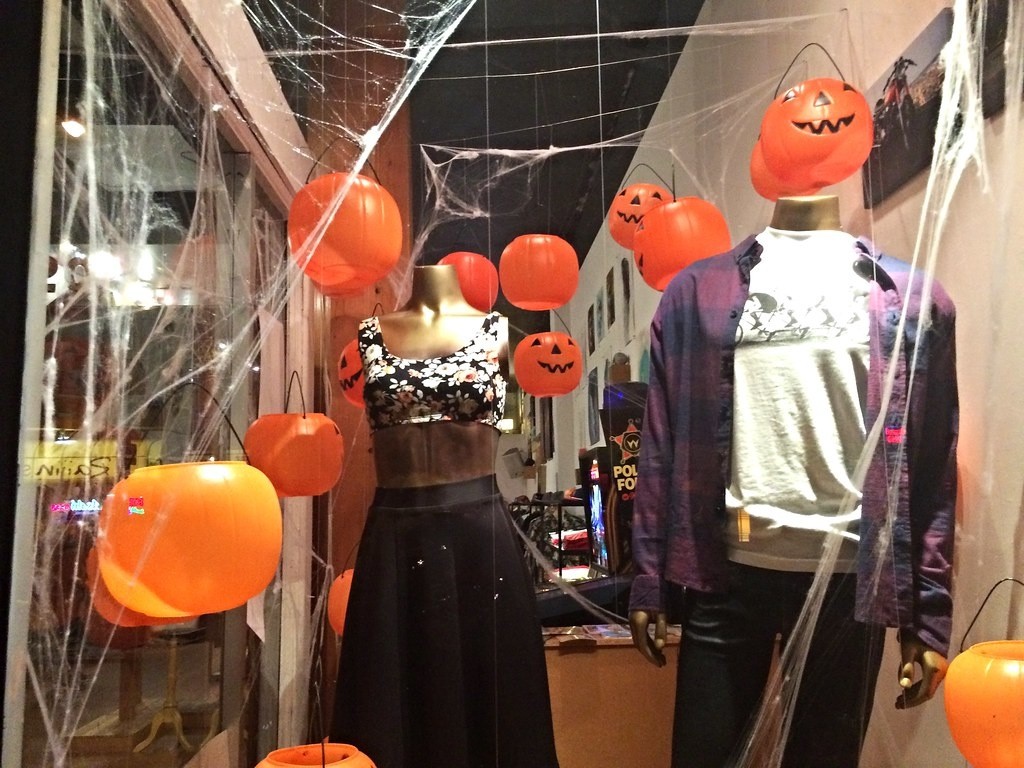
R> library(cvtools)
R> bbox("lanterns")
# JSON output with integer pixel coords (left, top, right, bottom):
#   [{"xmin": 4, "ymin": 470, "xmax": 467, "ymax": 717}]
[
  {"xmin": 85, "ymin": 541, "xmax": 197, "ymax": 627},
  {"xmin": 749, "ymin": 141, "xmax": 823, "ymax": 202},
  {"xmin": 943, "ymin": 641, "xmax": 1024, "ymax": 768},
  {"xmin": 435, "ymin": 251, "xmax": 500, "ymax": 314},
  {"xmin": 96, "ymin": 461, "xmax": 283, "ymax": 618},
  {"xmin": 288, "ymin": 173, "xmax": 403, "ymax": 283},
  {"xmin": 497, "ymin": 234, "xmax": 579, "ymax": 311},
  {"xmin": 758, "ymin": 78, "xmax": 876, "ymax": 189},
  {"xmin": 327, "ymin": 570, "xmax": 358, "ymax": 638},
  {"xmin": 243, "ymin": 412, "xmax": 345, "ymax": 496},
  {"xmin": 514, "ymin": 331, "xmax": 583, "ymax": 397},
  {"xmin": 336, "ymin": 338, "xmax": 367, "ymax": 407},
  {"xmin": 608, "ymin": 183, "xmax": 676, "ymax": 248},
  {"xmin": 253, "ymin": 742, "xmax": 380, "ymax": 768},
  {"xmin": 632, "ymin": 199, "xmax": 731, "ymax": 292},
  {"xmin": 307, "ymin": 278, "xmax": 369, "ymax": 292}
]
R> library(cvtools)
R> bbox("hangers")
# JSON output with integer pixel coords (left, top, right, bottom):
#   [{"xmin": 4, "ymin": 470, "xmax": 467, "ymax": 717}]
[{"xmin": 509, "ymin": 503, "xmax": 569, "ymax": 519}]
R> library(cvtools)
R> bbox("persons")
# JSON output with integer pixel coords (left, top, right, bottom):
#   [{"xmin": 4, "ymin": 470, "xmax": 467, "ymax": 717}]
[
  {"xmin": 628, "ymin": 197, "xmax": 959, "ymax": 768},
  {"xmin": 327, "ymin": 262, "xmax": 561, "ymax": 768}
]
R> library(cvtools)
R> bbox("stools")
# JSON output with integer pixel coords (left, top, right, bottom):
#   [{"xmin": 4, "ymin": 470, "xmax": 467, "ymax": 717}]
[{"xmin": 132, "ymin": 626, "xmax": 209, "ymax": 755}]
